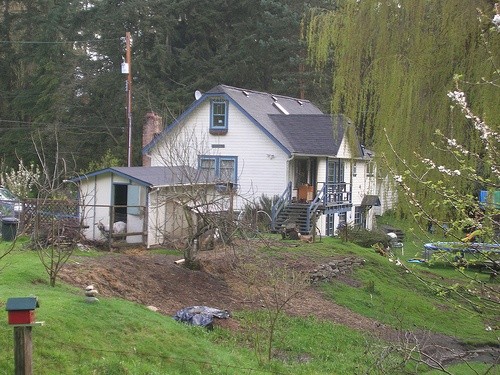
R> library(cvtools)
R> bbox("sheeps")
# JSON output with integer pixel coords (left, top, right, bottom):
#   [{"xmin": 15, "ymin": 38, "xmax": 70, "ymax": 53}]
[{"xmin": 94, "ymin": 218, "xmax": 127, "ymax": 242}]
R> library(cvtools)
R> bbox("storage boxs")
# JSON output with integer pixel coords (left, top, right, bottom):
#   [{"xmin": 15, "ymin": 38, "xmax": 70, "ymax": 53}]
[{"xmin": 299, "ymin": 186, "xmax": 314, "ymax": 200}]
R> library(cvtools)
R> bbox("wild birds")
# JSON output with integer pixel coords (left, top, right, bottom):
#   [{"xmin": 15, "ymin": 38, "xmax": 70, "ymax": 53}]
[{"xmin": 202, "ymin": 228, "xmax": 219, "ymax": 248}]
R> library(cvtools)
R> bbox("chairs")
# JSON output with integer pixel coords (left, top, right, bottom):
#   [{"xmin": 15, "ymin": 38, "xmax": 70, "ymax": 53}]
[{"xmin": 390, "ymin": 242, "xmax": 404, "ymax": 255}]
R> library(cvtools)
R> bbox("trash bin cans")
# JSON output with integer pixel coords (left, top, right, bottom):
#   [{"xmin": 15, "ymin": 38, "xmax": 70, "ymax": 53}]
[{"xmin": 1, "ymin": 216, "xmax": 19, "ymax": 241}]
